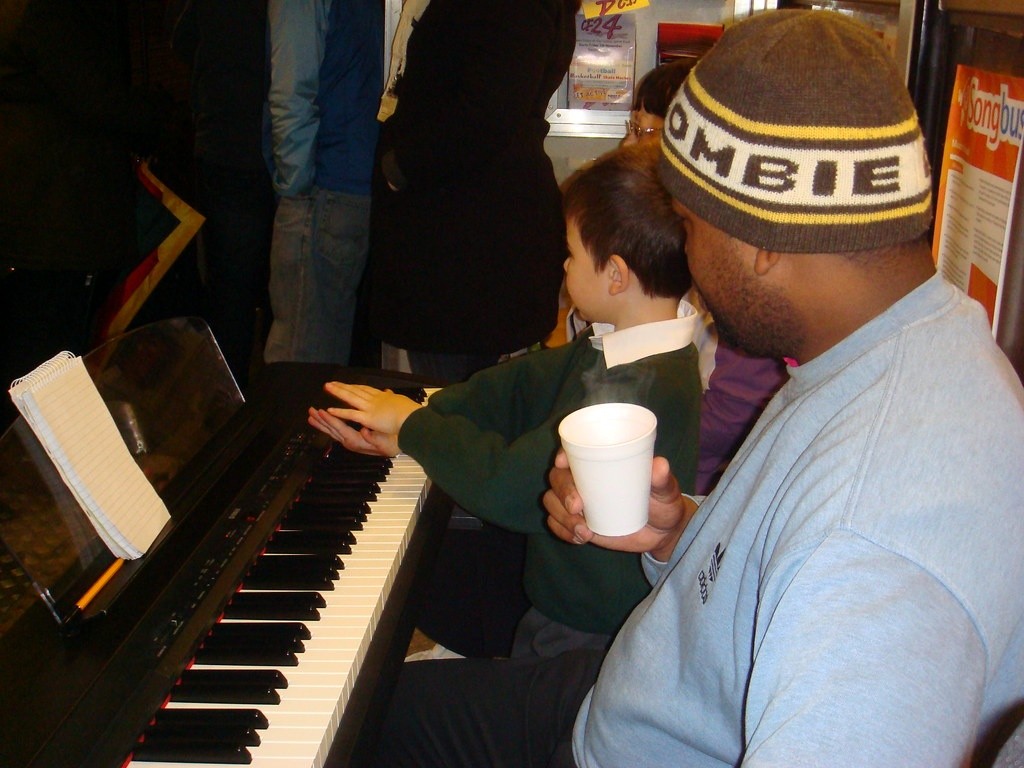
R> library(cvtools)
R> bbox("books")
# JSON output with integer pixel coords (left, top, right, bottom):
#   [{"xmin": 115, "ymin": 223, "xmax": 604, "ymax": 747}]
[{"xmin": 8, "ymin": 351, "xmax": 172, "ymax": 561}]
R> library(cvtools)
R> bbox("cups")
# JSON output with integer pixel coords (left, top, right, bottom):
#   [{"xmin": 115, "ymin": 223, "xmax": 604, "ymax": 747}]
[{"xmin": 558, "ymin": 402, "xmax": 656, "ymax": 536}]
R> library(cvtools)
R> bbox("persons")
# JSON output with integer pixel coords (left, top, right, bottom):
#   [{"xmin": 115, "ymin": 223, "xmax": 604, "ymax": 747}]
[
  {"xmin": 263, "ymin": 0, "xmax": 792, "ymax": 659},
  {"xmin": 367, "ymin": 9, "xmax": 1024, "ymax": 768}
]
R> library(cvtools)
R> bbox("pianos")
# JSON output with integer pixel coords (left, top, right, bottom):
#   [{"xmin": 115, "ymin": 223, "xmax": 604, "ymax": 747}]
[{"xmin": 1, "ymin": 312, "xmax": 457, "ymax": 768}]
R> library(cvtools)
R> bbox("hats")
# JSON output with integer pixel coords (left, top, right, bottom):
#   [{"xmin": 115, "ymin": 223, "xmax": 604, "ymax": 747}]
[{"xmin": 659, "ymin": 8, "xmax": 933, "ymax": 252}]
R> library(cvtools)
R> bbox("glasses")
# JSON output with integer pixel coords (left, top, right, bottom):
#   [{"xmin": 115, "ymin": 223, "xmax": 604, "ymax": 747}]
[{"xmin": 629, "ymin": 121, "xmax": 663, "ymax": 138}]
